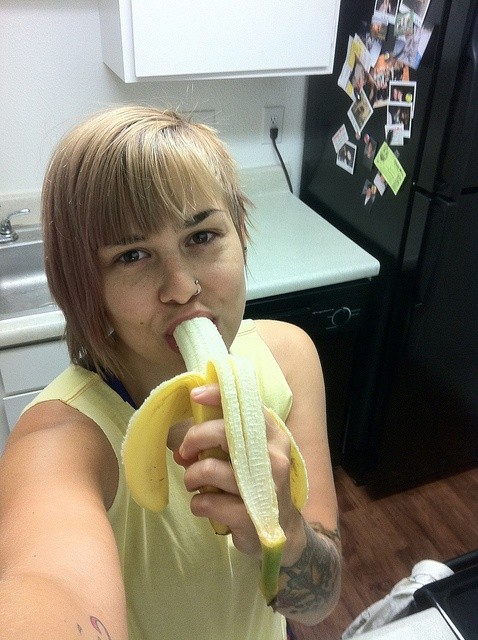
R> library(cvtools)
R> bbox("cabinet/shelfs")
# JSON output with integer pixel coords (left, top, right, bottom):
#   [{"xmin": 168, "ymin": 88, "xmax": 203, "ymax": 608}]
[
  {"xmin": 0, "ymin": 336, "xmax": 72, "ymax": 436},
  {"xmin": 97, "ymin": 0, "xmax": 342, "ymax": 85}
]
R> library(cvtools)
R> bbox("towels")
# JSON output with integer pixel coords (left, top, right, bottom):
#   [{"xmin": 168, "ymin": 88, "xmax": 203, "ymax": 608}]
[{"xmin": 337, "ymin": 551, "xmax": 456, "ymax": 631}]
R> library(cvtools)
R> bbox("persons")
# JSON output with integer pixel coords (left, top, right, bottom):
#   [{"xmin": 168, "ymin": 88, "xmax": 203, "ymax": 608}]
[{"xmin": 0, "ymin": 103, "xmax": 345, "ymax": 639}]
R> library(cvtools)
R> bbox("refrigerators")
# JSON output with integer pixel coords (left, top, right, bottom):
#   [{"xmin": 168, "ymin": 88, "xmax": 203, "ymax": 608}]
[{"xmin": 300, "ymin": 0, "xmax": 478, "ymax": 499}]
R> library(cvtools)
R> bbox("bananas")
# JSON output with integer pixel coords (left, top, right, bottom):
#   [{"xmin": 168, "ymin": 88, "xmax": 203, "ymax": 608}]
[{"xmin": 121, "ymin": 318, "xmax": 309, "ymax": 609}]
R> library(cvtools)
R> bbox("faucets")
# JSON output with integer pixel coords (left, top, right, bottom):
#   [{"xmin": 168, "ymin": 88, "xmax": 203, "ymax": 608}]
[{"xmin": 0, "ymin": 208, "xmax": 33, "ymax": 237}]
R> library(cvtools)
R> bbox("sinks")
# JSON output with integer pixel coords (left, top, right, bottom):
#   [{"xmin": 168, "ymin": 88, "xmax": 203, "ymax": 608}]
[{"xmin": 0, "ymin": 223, "xmax": 81, "ymax": 319}]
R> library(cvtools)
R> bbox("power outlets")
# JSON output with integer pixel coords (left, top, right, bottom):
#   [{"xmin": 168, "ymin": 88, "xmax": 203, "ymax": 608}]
[{"xmin": 262, "ymin": 107, "xmax": 284, "ymax": 144}]
